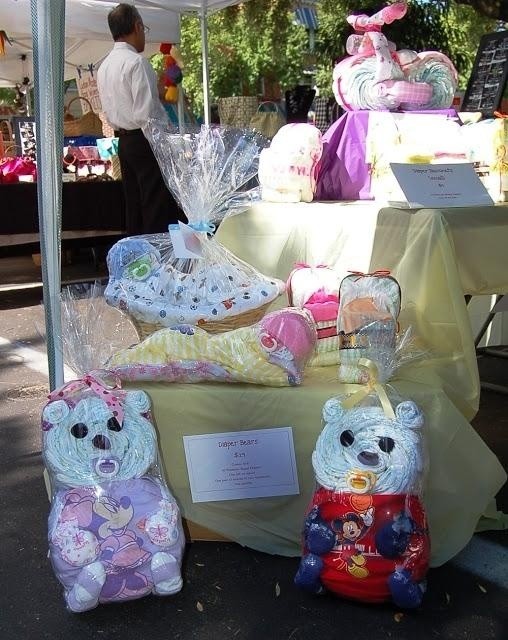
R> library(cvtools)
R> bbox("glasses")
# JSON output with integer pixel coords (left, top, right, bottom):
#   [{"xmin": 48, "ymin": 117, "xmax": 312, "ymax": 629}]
[{"xmin": 144, "ymin": 24, "xmax": 150, "ymax": 33}]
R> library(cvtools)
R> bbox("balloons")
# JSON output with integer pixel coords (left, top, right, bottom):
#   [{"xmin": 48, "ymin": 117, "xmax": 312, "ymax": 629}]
[{"xmin": 157, "ymin": 41, "xmax": 184, "ymax": 104}]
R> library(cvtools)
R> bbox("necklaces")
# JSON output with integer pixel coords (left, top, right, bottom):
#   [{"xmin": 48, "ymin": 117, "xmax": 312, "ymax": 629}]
[{"xmin": 62, "ymin": 155, "xmax": 77, "ymax": 165}]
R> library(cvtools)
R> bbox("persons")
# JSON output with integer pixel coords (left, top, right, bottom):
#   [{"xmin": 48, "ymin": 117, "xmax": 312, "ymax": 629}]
[{"xmin": 95, "ymin": 2, "xmax": 177, "ymax": 237}]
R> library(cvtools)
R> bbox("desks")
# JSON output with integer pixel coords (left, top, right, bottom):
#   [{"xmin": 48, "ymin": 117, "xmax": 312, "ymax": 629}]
[
  {"xmin": 1, "ymin": 178, "xmax": 131, "ymax": 313},
  {"xmin": 233, "ymin": 202, "xmax": 506, "ymax": 400}
]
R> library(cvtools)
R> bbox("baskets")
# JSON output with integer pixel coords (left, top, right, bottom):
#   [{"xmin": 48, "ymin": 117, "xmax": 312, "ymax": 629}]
[
  {"xmin": 337, "ymin": 271, "xmax": 401, "ymax": 383},
  {"xmin": 285, "ymin": 263, "xmax": 340, "ymax": 368},
  {"xmin": 107, "ymin": 293, "xmax": 282, "ymax": 340},
  {"xmin": 0, "ymin": 120, "xmax": 17, "ymax": 156},
  {"xmin": 63, "ymin": 97, "xmax": 102, "ymax": 137},
  {"xmin": 219, "ymin": 96, "xmax": 257, "ymax": 128}
]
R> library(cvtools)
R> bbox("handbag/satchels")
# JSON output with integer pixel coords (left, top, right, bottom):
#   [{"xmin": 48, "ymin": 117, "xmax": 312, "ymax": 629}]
[{"xmin": 250, "ymin": 101, "xmax": 286, "ymax": 138}]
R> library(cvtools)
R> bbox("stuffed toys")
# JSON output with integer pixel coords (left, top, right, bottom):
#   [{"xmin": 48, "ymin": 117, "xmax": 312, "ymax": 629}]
[
  {"xmin": 289, "ymin": 393, "xmax": 431, "ymax": 614},
  {"xmin": 256, "ymin": 123, "xmax": 325, "ymax": 204},
  {"xmin": 39, "ymin": 378, "xmax": 191, "ymax": 614},
  {"xmin": 106, "ymin": 235, "xmax": 254, "ymax": 310},
  {"xmin": 103, "ymin": 304, "xmax": 314, "ymax": 389}
]
